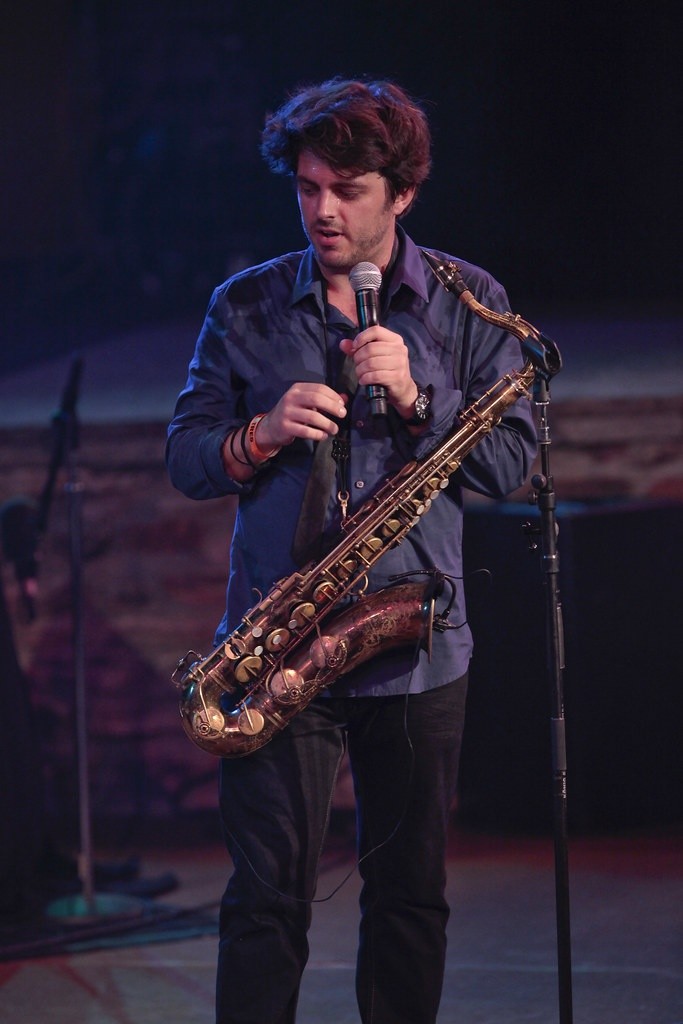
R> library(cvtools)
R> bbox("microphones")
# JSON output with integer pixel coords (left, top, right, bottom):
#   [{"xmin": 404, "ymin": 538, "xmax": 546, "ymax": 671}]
[
  {"xmin": 1, "ymin": 497, "xmax": 41, "ymax": 619},
  {"xmin": 349, "ymin": 261, "xmax": 389, "ymax": 418}
]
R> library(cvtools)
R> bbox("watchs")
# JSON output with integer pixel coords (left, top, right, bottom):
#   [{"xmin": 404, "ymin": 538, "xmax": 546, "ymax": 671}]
[{"xmin": 408, "ymin": 379, "xmax": 433, "ymax": 426}]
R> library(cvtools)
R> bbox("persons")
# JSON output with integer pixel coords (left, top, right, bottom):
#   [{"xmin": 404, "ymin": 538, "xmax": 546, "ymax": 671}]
[{"xmin": 165, "ymin": 78, "xmax": 538, "ymax": 1023}]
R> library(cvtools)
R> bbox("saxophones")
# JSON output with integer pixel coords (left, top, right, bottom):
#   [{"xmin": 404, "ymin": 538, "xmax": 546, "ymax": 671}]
[{"xmin": 169, "ymin": 249, "xmax": 566, "ymax": 756}]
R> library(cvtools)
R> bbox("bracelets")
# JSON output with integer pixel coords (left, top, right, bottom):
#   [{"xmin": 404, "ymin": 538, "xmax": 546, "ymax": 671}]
[
  {"xmin": 250, "ymin": 411, "xmax": 283, "ymax": 460},
  {"xmin": 229, "ymin": 423, "xmax": 271, "ymax": 471}
]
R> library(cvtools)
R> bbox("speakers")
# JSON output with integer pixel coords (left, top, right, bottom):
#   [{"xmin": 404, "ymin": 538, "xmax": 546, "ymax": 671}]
[{"xmin": 459, "ymin": 494, "xmax": 681, "ymax": 832}]
